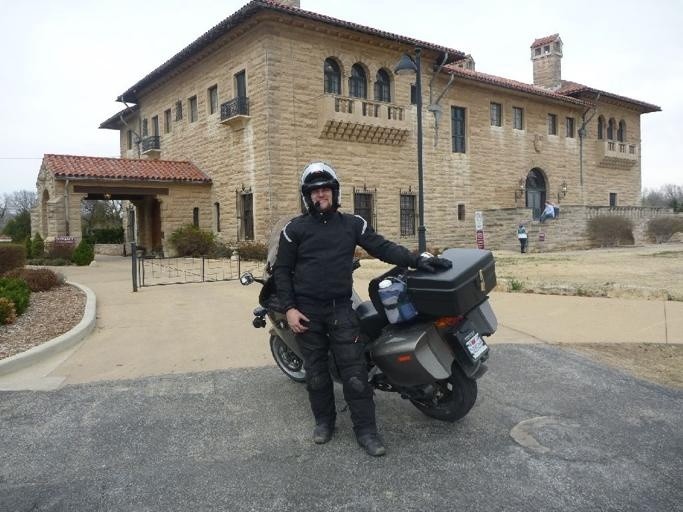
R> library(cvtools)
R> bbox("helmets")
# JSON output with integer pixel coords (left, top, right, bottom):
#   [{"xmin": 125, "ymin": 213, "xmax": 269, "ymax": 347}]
[{"xmin": 301, "ymin": 161, "xmax": 341, "ymax": 217}]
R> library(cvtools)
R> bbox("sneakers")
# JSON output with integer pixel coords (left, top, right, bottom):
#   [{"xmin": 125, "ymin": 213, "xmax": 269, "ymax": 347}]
[
  {"xmin": 353, "ymin": 425, "xmax": 386, "ymax": 456},
  {"xmin": 313, "ymin": 410, "xmax": 337, "ymax": 445}
]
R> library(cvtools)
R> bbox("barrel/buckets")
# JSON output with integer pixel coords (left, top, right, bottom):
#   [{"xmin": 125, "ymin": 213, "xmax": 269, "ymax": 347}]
[{"xmin": 377, "ymin": 280, "xmax": 419, "ymax": 325}]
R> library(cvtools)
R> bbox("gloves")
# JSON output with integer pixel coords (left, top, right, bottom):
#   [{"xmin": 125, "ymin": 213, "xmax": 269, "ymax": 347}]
[{"xmin": 414, "ymin": 252, "xmax": 453, "ymax": 274}]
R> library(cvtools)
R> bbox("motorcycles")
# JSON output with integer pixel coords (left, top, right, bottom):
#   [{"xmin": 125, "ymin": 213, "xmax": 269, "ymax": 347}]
[{"xmin": 239, "ymin": 247, "xmax": 496, "ymax": 423}]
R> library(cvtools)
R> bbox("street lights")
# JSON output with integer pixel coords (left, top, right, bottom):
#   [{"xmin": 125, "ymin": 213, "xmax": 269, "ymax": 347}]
[{"xmin": 392, "ymin": 47, "xmax": 426, "ymax": 253}]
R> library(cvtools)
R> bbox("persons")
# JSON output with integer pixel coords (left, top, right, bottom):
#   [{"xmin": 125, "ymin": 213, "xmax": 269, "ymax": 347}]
[
  {"xmin": 517, "ymin": 223, "xmax": 529, "ymax": 253},
  {"xmin": 272, "ymin": 163, "xmax": 452, "ymax": 458},
  {"xmin": 540, "ymin": 201, "xmax": 555, "ymax": 224}
]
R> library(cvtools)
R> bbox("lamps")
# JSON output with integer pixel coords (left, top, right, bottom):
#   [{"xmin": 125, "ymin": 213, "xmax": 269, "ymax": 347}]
[
  {"xmin": 514, "ymin": 176, "xmax": 524, "ymax": 202},
  {"xmin": 557, "ymin": 179, "xmax": 568, "ymax": 202}
]
